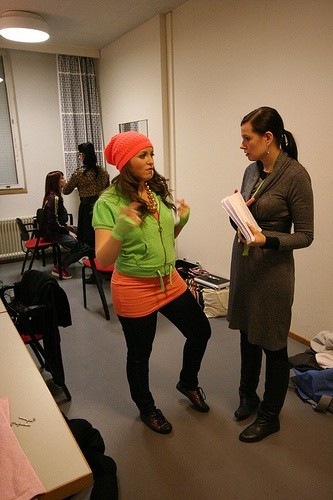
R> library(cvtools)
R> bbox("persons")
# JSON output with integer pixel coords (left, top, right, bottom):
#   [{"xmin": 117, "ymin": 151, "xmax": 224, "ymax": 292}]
[
  {"xmin": 41, "ymin": 171, "xmax": 89, "ymax": 279},
  {"xmin": 226, "ymin": 107, "xmax": 315, "ymax": 443},
  {"xmin": 60, "ymin": 143, "xmax": 110, "ymax": 264},
  {"xmin": 91, "ymin": 132, "xmax": 212, "ymax": 435}
]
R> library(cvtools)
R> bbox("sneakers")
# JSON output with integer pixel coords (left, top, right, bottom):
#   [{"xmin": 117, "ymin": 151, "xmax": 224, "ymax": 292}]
[
  {"xmin": 140, "ymin": 405, "xmax": 172, "ymax": 434},
  {"xmin": 176, "ymin": 381, "xmax": 210, "ymax": 412}
]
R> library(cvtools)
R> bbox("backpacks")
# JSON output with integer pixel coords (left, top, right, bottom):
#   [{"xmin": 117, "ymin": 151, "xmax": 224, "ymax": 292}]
[{"xmin": 66, "ymin": 418, "xmax": 119, "ymax": 500}]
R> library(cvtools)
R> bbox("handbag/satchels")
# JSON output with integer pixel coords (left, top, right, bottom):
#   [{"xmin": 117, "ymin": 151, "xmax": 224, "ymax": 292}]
[
  {"xmin": 288, "ymin": 348, "xmax": 322, "ymax": 371},
  {"xmin": 289, "ymin": 367, "xmax": 333, "ymax": 413}
]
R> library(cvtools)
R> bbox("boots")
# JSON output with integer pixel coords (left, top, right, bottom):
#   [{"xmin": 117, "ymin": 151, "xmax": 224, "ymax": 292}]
[{"xmin": 235, "ymin": 358, "xmax": 288, "ymax": 443}]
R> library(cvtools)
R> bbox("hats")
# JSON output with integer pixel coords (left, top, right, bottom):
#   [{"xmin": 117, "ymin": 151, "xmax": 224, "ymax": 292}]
[{"xmin": 105, "ymin": 131, "xmax": 154, "ymax": 173}]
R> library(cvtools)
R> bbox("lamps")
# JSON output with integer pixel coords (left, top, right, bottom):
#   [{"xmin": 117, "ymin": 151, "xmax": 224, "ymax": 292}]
[{"xmin": 0, "ymin": 10, "xmax": 51, "ymax": 43}]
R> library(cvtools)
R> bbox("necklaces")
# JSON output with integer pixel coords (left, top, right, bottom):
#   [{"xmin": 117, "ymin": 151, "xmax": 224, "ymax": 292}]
[{"xmin": 144, "ymin": 182, "xmax": 157, "ymax": 213}]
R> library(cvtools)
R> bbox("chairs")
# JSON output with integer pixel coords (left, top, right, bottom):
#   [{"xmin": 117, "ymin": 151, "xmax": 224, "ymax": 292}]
[{"xmin": 0, "ymin": 209, "xmax": 114, "ymax": 401}]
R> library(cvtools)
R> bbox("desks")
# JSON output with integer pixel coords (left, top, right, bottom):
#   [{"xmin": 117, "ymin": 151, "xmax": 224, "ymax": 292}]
[{"xmin": 0, "ymin": 297, "xmax": 94, "ymax": 500}]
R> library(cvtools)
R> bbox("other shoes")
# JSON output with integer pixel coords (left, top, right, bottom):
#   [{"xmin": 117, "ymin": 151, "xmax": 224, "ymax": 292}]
[
  {"xmin": 51, "ymin": 265, "xmax": 72, "ymax": 279},
  {"xmin": 84, "ymin": 274, "xmax": 102, "ymax": 283}
]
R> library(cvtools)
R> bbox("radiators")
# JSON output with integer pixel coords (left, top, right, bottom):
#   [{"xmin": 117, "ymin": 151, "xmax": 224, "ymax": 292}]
[{"xmin": 0, "ymin": 215, "xmax": 53, "ymax": 262}]
